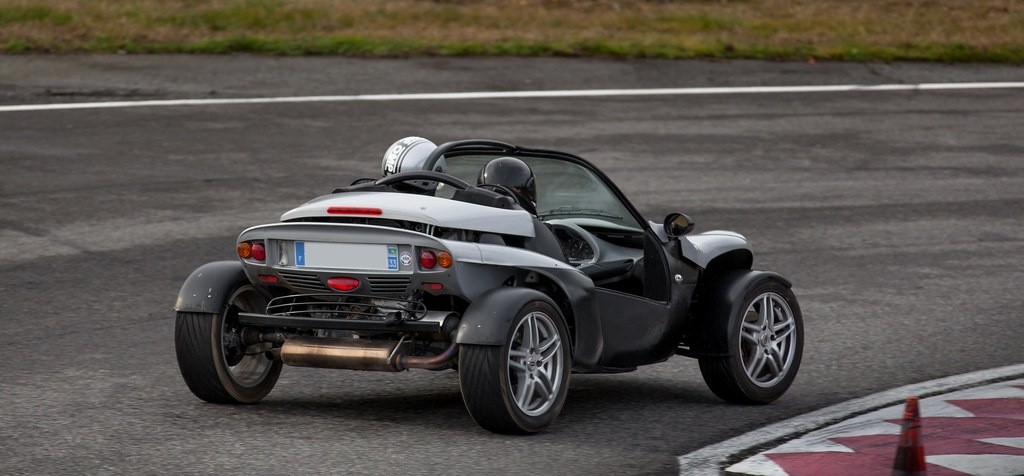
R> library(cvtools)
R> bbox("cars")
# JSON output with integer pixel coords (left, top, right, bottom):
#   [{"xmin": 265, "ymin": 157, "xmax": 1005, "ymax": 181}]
[{"xmin": 174, "ymin": 138, "xmax": 805, "ymax": 435}]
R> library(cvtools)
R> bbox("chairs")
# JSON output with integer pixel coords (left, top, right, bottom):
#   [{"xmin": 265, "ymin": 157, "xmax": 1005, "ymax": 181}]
[{"xmin": 511, "ymin": 202, "xmax": 567, "ymax": 263}]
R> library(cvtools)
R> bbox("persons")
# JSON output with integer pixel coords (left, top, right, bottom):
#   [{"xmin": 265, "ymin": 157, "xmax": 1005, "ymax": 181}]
[
  {"xmin": 477, "ymin": 157, "xmax": 539, "ymax": 214},
  {"xmin": 381, "ymin": 135, "xmax": 445, "ymax": 193}
]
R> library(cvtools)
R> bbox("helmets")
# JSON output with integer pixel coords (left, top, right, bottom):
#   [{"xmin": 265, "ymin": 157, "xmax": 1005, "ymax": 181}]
[
  {"xmin": 476, "ymin": 158, "xmax": 538, "ymax": 214},
  {"xmin": 380, "ymin": 136, "xmax": 446, "ymax": 196}
]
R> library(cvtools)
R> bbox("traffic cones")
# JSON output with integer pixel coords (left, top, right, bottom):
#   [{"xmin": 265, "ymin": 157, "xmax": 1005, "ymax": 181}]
[{"xmin": 893, "ymin": 396, "xmax": 927, "ymax": 472}]
